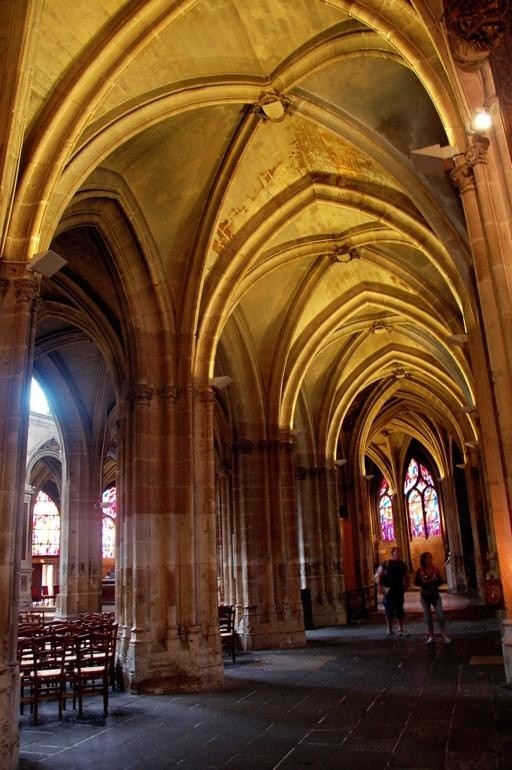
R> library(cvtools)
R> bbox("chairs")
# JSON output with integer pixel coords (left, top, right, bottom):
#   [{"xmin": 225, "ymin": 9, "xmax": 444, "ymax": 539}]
[
  {"xmin": 218, "ymin": 604, "xmax": 238, "ymax": 664},
  {"xmin": 17, "ymin": 579, "xmax": 119, "ymax": 720}
]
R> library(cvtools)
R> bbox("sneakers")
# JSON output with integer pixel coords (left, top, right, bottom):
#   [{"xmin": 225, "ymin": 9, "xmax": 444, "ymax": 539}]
[
  {"xmin": 424, "ymin": 636, "xmax": 435, "ymax": 645},
  {"xmin": 385, "ymin": 628, "xmax": 404, "ymax": 635},
  {"xmin": 442, "ymin": 635, "xmax": 452, "ymax": 644}
]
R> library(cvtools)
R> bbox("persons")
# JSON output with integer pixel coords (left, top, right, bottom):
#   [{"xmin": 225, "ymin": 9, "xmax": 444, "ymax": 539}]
[
  {"xmin": 413, "ymin": 551, "xmax": 452, "ymax": 645},
  {"xmin": 374, "ymin": 546, "xmax": 411, "ymax": 635}
]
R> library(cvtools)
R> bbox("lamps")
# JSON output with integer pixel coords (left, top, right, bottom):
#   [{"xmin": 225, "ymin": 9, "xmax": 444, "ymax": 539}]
[
  {"xmin": 365, "ymin": 475, "xmax": 374, "ymax": 480},
  {"xmin": 335, "ymin": 458, "xmax": 347, "ymax": 466},
  {"xmin": 213, "ymin": 376, "xmax": 233, "ymax": 389},
  {"xmin": 464, "ymin": 108, "xmax": 492, "ymax": 174},
  {"xmin": 27, "ymin": 250, "xmax": 68, "ymax": 279}
]
